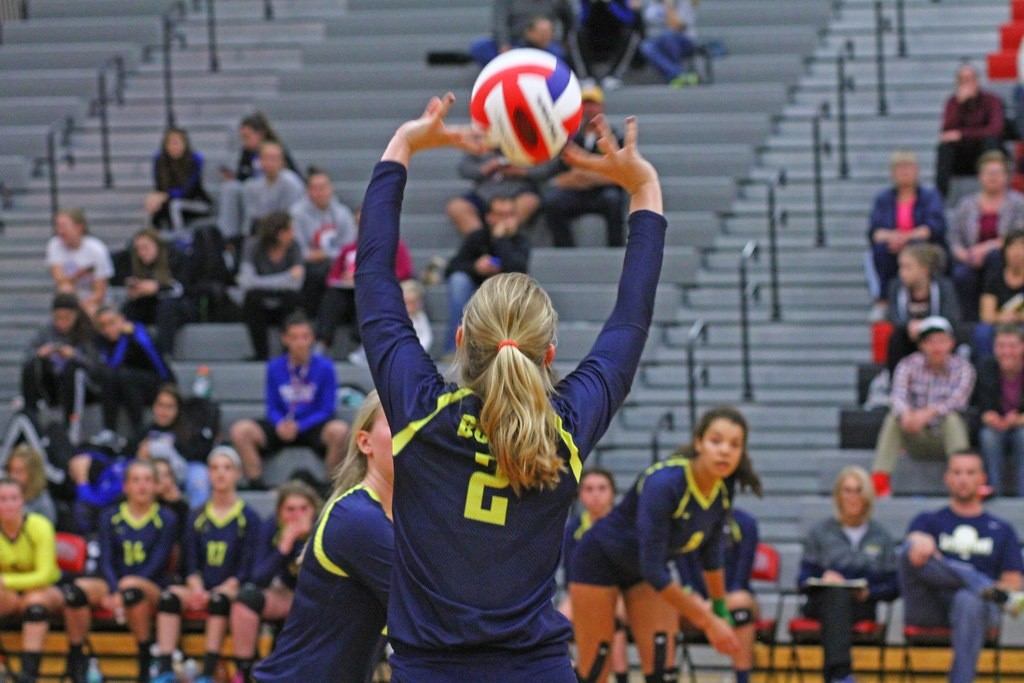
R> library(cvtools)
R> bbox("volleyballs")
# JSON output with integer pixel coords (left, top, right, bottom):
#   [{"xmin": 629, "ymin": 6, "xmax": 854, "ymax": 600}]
[{"xmin": 470, "ymin": 47, "xmax": 584, "ymax": 168}]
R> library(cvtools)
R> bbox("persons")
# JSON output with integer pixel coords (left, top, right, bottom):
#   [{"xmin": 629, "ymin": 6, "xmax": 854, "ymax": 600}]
[
  {"xmin": 1, "ymin": 0, "xmax": 1024, "ymax": 683},
  {"xmin": 354, "ymin": 93, "xmax": 667, "ymax": 682}
]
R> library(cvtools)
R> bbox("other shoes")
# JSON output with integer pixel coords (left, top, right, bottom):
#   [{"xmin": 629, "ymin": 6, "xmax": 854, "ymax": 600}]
[
  {"xmin": 601, "ymin": 75, "xmax": 624, "ymax": 92},
  {"xmin": 869, "ymin": 303, "xmax": 889, "ymax": 324},
  {"xmin": 242, "ymin": 352, "xmax": 271, "ymax": 362},
  {"xmin": 1002, "ymin": 591, "xmax": 1024, "ymax": 617},
  {"xmin": 90, "ymin": 429, "xmax": 119, "ymax": 447}
]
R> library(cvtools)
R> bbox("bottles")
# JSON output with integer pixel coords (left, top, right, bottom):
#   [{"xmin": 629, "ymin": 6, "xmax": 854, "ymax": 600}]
[{"xmin": 192, "ymin": 367, "xmax": 214, "ymax": 397}]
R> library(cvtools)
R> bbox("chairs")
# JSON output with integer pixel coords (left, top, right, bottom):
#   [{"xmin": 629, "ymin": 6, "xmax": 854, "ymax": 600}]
[{"xmin": 0, "ymin": 533, "xmax": 1004, "ymax": 683}]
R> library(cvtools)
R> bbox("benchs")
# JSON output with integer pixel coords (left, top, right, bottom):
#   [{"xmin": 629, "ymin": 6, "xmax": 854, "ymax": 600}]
[{"xmin": 0, "ymin": 0, "xmax": 1024, "ymax": 643}]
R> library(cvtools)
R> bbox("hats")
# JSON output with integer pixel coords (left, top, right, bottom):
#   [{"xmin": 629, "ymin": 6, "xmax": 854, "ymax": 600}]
[
  {"xmin": 917, "ymin": 315, "xmax": 955, "ymax": 338},
  {"xmin": 979, "ymin": 150, "xmax": 1006, "ymax": 164},
  {"xmin": 582, "ymin": 86, "xmax": 603, "ymax": 105}
]
